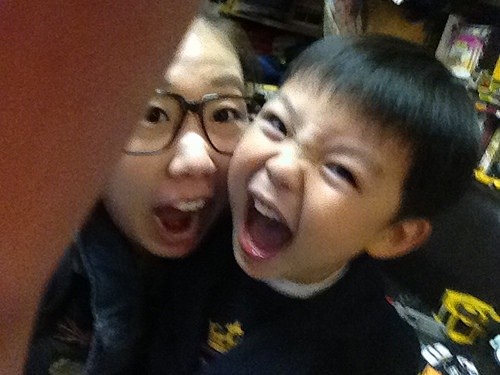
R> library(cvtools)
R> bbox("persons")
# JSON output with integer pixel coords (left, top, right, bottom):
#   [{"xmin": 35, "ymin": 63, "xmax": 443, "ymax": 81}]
[
  {"xmin": 1, "ymin": 0, "xmax": 248, "ymax": 375},
  {"xmin": 171, "ymin": 31, "xmax": 484, "ymax": 375}
]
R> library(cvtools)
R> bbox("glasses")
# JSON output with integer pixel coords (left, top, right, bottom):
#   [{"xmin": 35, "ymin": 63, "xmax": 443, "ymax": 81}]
[{"xmin": 121, "ymin": 92, "xmax": 255, "ymax": 156}]
